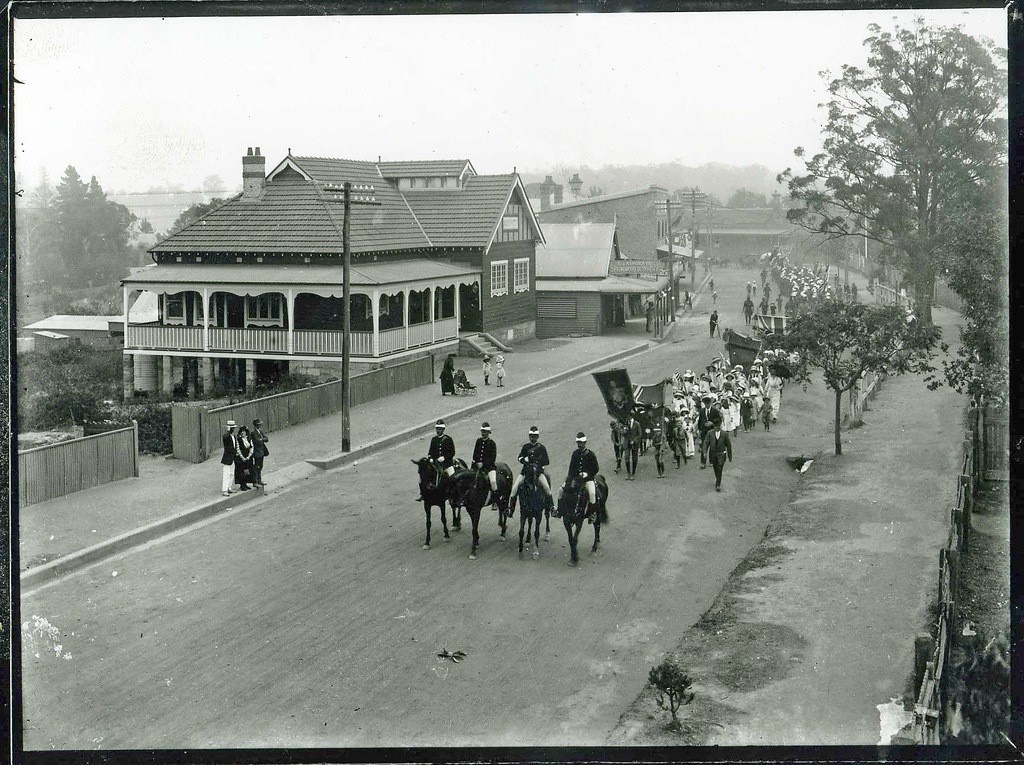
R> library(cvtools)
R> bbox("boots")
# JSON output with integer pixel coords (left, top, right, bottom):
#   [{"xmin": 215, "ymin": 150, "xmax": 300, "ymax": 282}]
[
  {"xmin": 548, "ymin": 493, "xmax": 557, "ymax": 517},
  {"xmin": 493, "ymin": 489, "xmax": 506, "ymax": 513},
  {"xmin": 588, "ymin": 501, "xmax": 596, "ymax": 523},
  {"xmin": 504, "ymin": 494, "xmax": 518, "ymax": 517}
]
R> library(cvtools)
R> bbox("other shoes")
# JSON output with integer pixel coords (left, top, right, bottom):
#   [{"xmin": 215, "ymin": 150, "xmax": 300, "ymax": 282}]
[
  {"xmin": 497, "ymin": 384, "xmax": 503, "ymax": 387},
  {"xmin": 415, "ymin": 494, "xmax": 422, "ymax": 501},
  {"xmin": 451, "ymin": 392, "xmax": 458, "ymax": 395},
  {"xmin": 614, "ymin": 459, "xmax": 724, "ymax": 492},
  {"xmin": 223, "ymin": 481, "xmax": 268, "ymax": 496},
  {"xmin": 442, "ymin": 394, "xmax": 446, "ymax": 396},
  {"xmin": 485, "ymin": 383, "xmax": 490, "ymax": 385}
]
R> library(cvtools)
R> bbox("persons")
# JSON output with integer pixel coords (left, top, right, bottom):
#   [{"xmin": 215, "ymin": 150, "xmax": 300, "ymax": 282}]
[
  {"xmin": 610, "ymin": 253, "xmax": 858, "ymax": 492},
  {"xmin": 503, "ymin": 426, "xmax": 556, "ymax": 517},
  {"xmin": 552, "ymin": 432, "xmax": 599, "ymax": 524},
  {"xmin": 496, "ymin": 355, "xmax": 506, "ymax": 387},
  {"xmin": 220, "ymin": 418, "xmax": 238, "ymax": 496},
  {"xmin": 471, "ymin": 421, "xmax": 497, "ymax": 511},
  {"xmin": 251, "ymin": 418, "xmax": 269, "ymax": 488},
  {"xmin": 439, "ymin": 354, "xmax": 458, "ymax": 396},
  {"xmin": 414, "ymin": 419, "xmax": 455, "ymax": 502},
  {"xmin": 234, "ymin": 426, "xmax": 254, "ymax": 491},
  {"xmin": 482, "ymin": 355, "xmax": 492, "ymax": 386}
]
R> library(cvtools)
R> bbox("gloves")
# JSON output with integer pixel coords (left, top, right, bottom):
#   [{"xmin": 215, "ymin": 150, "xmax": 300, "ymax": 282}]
[
  {"xmin": 579, "ymin": 472, "xmax": 588, "ymax": 478},
  {"xmin": 525, "ymin": 456, "xmax": 529, "ymax": 462},
  {"xmin": 429, "ymin": 459, "xmax": 433, "ymax": 463},
  {"xmin": 477, "ymin": 462, "xmax": 483, "ymax": 468},
  {"xmin": 437, "ymin": 456, "xmax": 444, "ymax": 462}
]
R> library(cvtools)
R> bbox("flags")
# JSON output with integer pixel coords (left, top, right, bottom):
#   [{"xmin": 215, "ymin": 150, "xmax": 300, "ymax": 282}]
[{"xmin": 632, "ymin": 380, "xmax": 664, "ymax": 405}]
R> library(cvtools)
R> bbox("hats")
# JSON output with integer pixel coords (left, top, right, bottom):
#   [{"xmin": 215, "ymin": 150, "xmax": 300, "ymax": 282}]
[
  {"xmin": 496, "ymin": 355, "xmax": 504, "ymax": 362},
  {"xmin": 575, "ymin": 431, "xmax": 587, "ymax": 443},
  {"xmin": 226, "ymin": 420, "xmax": 238, "ymax": 427},
  {"xmin": 529, "ymin": 426, "xmax": 539, "ymax": 435},
  {"xmin": 610, "ymin": 354, "xmax": 777, "ymax": 432},
  {"xmin": 480, "ymin": 421, "xmax": 492, "ymax": 434},
  {"xmin": 482, "ymin": 355, "xmax": 490, "ymax": 361},
  {"xmin": 435, "ymin": 419, "xmax": 445, "ymax": 429},
  {"xmin": 448, "ymin": 353, "xmax": 457, "ymax": 357},
  {"xmin": 252, "ymin": 418, "xmax": 263, "ymax": 426}
]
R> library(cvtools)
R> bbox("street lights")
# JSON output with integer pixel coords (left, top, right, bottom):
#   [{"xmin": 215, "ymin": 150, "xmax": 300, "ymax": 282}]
[
  {"xmin": 317, "ymin": 182, "xmax": 383, "ymax": 452},
  {"xmin": 654, "ymin": 199, "xmax": 682, "ymax": 323},
  {"xmin": 682, "ymin": 192, "xmax": 708, "ymax": 282}
]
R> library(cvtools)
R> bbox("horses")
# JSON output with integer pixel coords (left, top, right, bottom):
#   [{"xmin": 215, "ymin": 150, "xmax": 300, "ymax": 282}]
[
  {"xmin": 445, "ymin": 462, "xmax": 513, "ymax": 560},
  {"xmin": 559, "ymin": 473, "xmax": 609, "ymax": 565},
  {"xmin": 517, "ymin": 462, "xmax": 552, "ymax": 560},
  {"xmin": 411, "ymin": 457, "xmax": 469, "ymax": 550}
]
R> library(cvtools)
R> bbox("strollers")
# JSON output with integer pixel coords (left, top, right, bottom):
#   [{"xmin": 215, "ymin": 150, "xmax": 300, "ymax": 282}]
[{"xmin": 453, "ymin": 369, "xmax": 478, "ymax": 397}]
